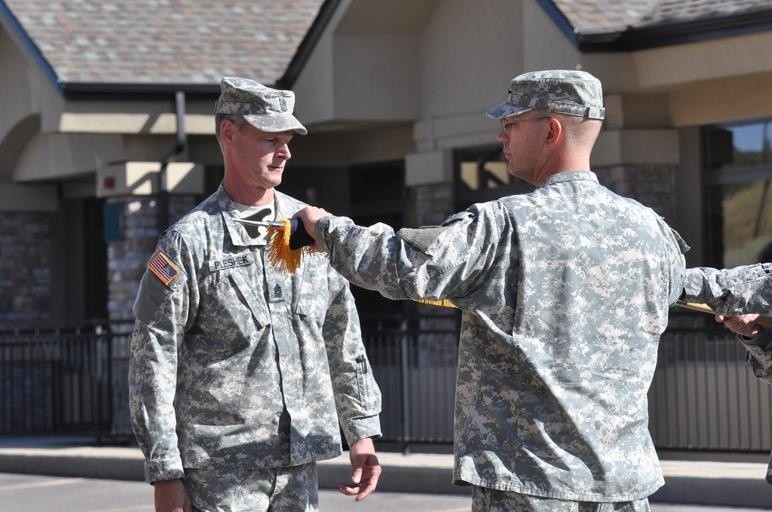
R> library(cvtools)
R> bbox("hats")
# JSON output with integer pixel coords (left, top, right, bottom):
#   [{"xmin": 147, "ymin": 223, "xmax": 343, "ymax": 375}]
[
  {"xmin": 485, "ymin": 69, "xmax": 606, "ymax": 120},
  {"xmin": 215, "ymin": 76, "xmax": 308, "ymax": 136}
]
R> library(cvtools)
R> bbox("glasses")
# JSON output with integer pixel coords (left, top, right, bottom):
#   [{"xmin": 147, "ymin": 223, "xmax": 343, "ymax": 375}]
[{"xmin": 497, "ymin": 116, "xmax": 552, "ymax": 132}]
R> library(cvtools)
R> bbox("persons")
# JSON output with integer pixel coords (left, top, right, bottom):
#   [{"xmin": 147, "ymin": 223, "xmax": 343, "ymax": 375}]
[
  {"xmin": 680, "ymin": 262, "xmax": 772, "ymax": 485},
  {"xmin": 292, "ymin": 68, "xmax": 692, "ymax": 512},
  {"xmin": 127, "ymin": 77, "xmax": 382, "ymax": 512}
]
